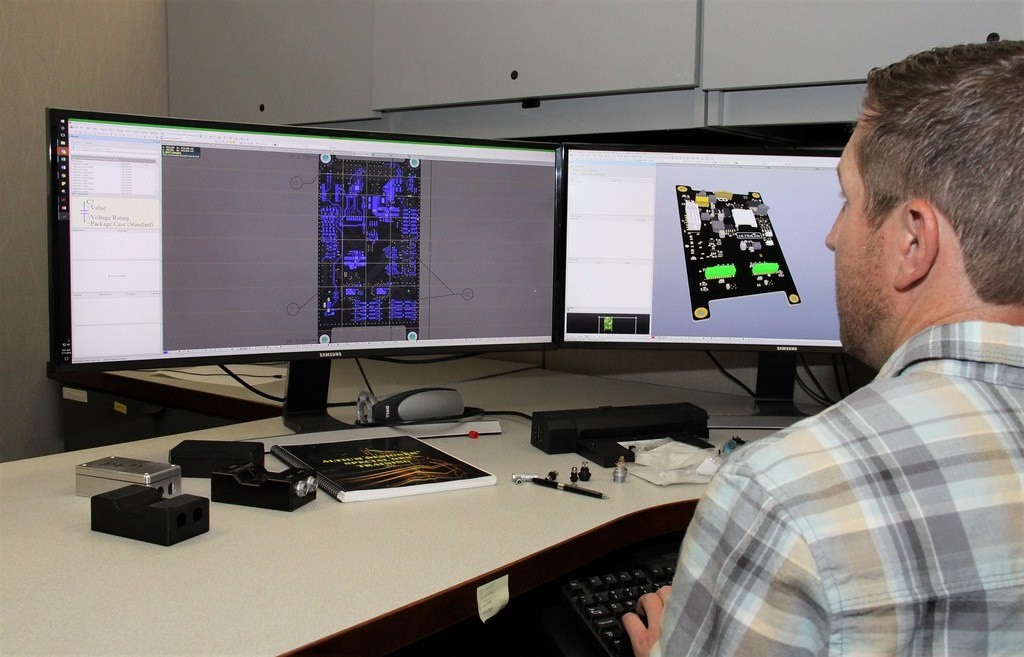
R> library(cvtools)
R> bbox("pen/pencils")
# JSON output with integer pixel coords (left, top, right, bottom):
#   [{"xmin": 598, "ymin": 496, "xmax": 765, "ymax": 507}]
[{"xmin": 532, "ymin": 477, "xmax": 610, "ymax": 500}]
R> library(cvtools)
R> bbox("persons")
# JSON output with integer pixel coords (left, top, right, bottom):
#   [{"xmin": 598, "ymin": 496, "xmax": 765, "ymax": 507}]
[{"xmin": 621, "ymin": 39, "xmax": 1024, "ymax": 656}]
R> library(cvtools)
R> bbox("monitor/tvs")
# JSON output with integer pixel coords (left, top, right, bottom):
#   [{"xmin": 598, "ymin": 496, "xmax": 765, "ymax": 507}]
[
  {"xmin": 45, "ymin": 108, "xmax": 563, "ymax": 454},
  {"xmin": 561, "ymin": 143, "xmax": 847, "ymax": 430}
]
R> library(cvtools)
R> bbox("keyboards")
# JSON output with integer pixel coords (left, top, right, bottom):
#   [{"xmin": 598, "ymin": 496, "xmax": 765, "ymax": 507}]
[{"xmin": 561, "ymin": 561, "xmax": 677, "ymax": 657}]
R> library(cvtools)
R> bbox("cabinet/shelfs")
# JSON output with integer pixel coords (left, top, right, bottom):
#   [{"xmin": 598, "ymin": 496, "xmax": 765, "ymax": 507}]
[{"xmin": 166, "ymin": 0, "xmax": 1024, "ymax": 164}]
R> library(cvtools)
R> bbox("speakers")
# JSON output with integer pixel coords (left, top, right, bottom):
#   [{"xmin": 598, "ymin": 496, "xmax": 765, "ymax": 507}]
[{"xmin": 356, "ymin": 387, "xmax": 465, "ymax": 424}]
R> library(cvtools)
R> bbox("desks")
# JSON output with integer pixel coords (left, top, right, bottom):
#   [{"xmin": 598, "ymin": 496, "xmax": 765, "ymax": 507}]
[
  {"xmin": 45, "ymin": 357, "xmax": 537, "ymax": 452},
  {"xmin": 0, "ymin": 367, "xmax": 823, "ymax": 657}
]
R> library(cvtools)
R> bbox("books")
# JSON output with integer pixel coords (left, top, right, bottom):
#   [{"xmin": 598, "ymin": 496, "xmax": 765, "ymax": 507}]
[{"xmin": 270, "ymin": 433, "xmax": 500, "ymax": 504}]
